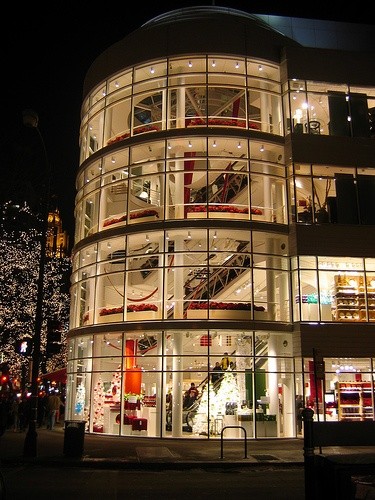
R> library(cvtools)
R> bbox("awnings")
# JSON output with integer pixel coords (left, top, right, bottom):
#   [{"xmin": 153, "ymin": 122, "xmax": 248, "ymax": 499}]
[{"xmin": 37, "ymin": 367, "xmax": 67, "ymax": 384}]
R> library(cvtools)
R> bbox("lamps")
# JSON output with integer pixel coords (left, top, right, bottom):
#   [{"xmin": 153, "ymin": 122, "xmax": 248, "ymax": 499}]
[
  {"xmin": 213, "ymin": 230, "xmax": 217, "ymax": 239},
  {"xmin": 259, "ymin": 144, "xmax": 265, "ymax": 152},
  {"xmin": 168, "ymin": 142, "xmax": 172, "ymax": 149},
  {"xmin": 187, "ymin": 231, "xmax": 192, "ymax": 239},
  {"xmin": 292, "ymin": 83, "xmax": 323, "ymax": 104},
  {"xmin": 188, "ymin": 60, "xmax": 193, "ymax": 68},
  {"xmin": 98, "ymin": 163, "xmax": 102, "ymax": 171},
  {"xmin": 212, "ymin": 139, "xmax": 217, "ymax": 148},
  {"xmin": 235, "ymin": 61, "xmax": 240, "ymax": 69},
  {"xmin": 79, "ymin": 332, "xmax": 245, "ymax": 345},
  {"xmin": 212, "ymin": 60, "xmax": 216, "ymax": 68},
  {"xmin": 257, "ymin": 64, "xmax": 258, "ymax": 72},
  {"xmin": 189, "ymin": 140, "xmax": 193, "ymax": 147},
  {"xmin": 237, "ymin": 141, "xmax": 241, "ymax": 149},
  {"xmin": 115, "ymin": 81, "xmax": 120, "ymax": 88},
  {"xmin": 81, "ymin": 232, "xmax": 170, "ymax": 262},
  {"xmin": 148, "ymin": 145, "xmax": 153, "ymax": 152},
  {"xmin": 111, "ymin": 156, "xmax": 116, "ymax": 164},
  {"xmin": 169, "ymin": 63, "xmax": 173, "ymax": 70},
  {"xmin": 103, "ymin": 90, "xmax": 107, "ymax": 96},
  {"xmin": 150, "ymin": 67, "xmax": 155, "ymax": 74}
]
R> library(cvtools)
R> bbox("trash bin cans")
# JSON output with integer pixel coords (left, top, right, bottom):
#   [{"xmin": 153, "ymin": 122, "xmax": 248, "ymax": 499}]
[{"xmin": 63, "ymin": 420, "xmax": 87, "ymax": 454}]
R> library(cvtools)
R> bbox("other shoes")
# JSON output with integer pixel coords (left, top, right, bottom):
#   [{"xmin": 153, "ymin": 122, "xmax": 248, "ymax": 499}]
[
  {"xmin": 47, "ymin": 425, "xmax": 53, "ymax": 430},
  {"xmin": 10, "ymin": 427, "xmax": 16, "ymax": 432},
  {"xmin": 19, "ymin": 429, "xmax": 26, "ymax": 433}
]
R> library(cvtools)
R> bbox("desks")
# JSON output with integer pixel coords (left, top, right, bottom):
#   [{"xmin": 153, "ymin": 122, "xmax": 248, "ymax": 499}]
[
  {"xmin": 142, "ymin": 406, "xmax": 156, "ymax": 437},
  {"xmin": 112, "ymin": 423, "xmax": 133, "ymax": 435},
  {"xmin": 238, "ymin": 414, "xmax": 278, "ymax": 437},
  {"xmin": 104, "ymin": 405, "xmax": 120, "ymax": 432},
  {"xmin": 223, "ymin": 414, "xmax": 239, "ymax": 437}
]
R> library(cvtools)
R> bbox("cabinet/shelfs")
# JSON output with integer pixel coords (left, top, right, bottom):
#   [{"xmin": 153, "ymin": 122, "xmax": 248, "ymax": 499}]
[
  {"xmin": 330, "ymin": 274, "xmax": 375, "ymax": 321},
  {"xmin": 336, "ymin": 381, "xmax": 375, "ymax": 422}
]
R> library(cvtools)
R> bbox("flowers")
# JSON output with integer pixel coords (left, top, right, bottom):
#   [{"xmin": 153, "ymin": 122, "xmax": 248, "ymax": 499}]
[
  {"xmin": 187, "ymin": 302, "xmax": 266, "ymax": 312},
  {"xmin": 108, "ymin": 124, "xmax": 160, "ymax": 146},
  {"xmin": 100, "ymin": 209, "xmax": 160, "ymax": 227},
  {"xmin": 186, "ymin": 205, "xmax": 262, "ymax": 215},
  {"xmin": 188, "ymin": 117, "xmax": 262, "ymax": 130},
  {"xmin": 82, "ymin": 303, "xmax": 159, "ymax": 321}
]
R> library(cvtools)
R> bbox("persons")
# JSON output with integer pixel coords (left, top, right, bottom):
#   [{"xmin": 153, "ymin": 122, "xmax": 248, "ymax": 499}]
[
  {"xmin": 166, "ymin": 383, "xmax": 199, "ymax": 417},
  {"xmin": 0, "ymin": 378, "xmax": 64, "ymax": 434},
  {"xmin": 193, "ymin": 165, "xmax": 236, "ymax": 202},
  {"xmin": 210, "ymin": 352, "xmax": 236, "ymax": 388},
  {"xmin": 301, "ymin": 196, "xmax": 340, "ymax": 223},
  {"xmin": 295, "ymin": 394, "xmax": 315, "ymax": 435},
  {"xmin": 235, "ymin": 241, "xmax": 246, "ymax": 252},
  {"xmin": 185, "ymin": 280, "xmax": 193, "ymax": 295}
]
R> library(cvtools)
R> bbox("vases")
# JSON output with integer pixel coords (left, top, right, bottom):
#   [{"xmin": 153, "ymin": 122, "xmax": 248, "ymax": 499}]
[
  {"xmin": 101, "ymin": 215, "xmax": 156, "ymax": 231},
  {"xmin": 85, "ymin": 311, "xmax": 157, "ymax": 323},
  {"xmin": 186, "ymin": 212, "xmax": 265, "ymax": 222},
  {"xmin": 124, "ymin": 129, "xmax": 157, "ymax": 140},
  {"xmin": 187, "ymin": 125, "xmax": 260, "ymax": 132},
  {"xmin": 186, "ymin": 309, "xmax": 268, "ymax": 321}
]
